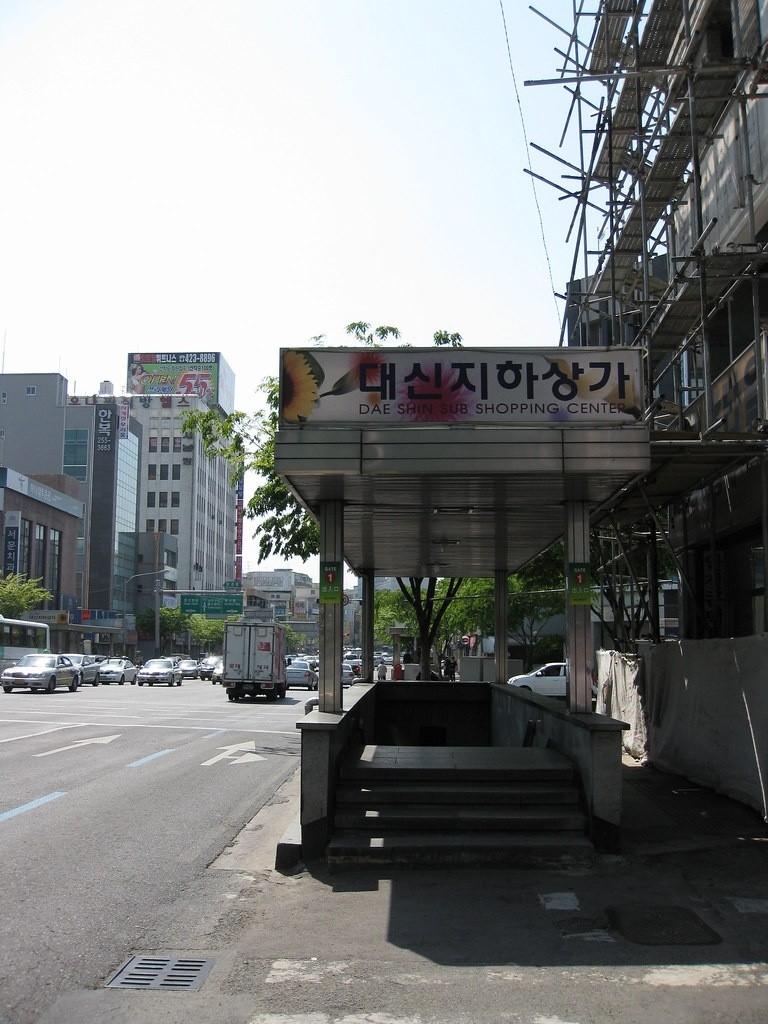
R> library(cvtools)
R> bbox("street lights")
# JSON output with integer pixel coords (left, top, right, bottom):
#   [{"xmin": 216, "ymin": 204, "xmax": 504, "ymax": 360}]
[{"xmin": 121, "ymin": 569, "xmax": 171, "ymax": 656}]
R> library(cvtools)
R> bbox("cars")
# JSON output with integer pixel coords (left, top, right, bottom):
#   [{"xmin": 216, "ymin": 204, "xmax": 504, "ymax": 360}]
[
  {"xmin": 97, "ymin": 658, "xmax": 136, "ymax": 686},
  {"xmin": 211, "ymin": 660, "xmax": 224, "ymax": 685},
  {"xmin": 508, "ymin": 661, "xmax": 566, "ymax": 699},
  {"xmin": 283, "ymin": 645, "xmax": 406, "ymax": 688},
  {"xmin": 175, "ymin": 660, "xmax": 200, "ymax": 680},
  {"xmin": 60, "ymin": 653, "xmax": 100, "ymax": 687},
  {"xmin": 198, "ymin": 657, "xmax": 222, "ymax": 680},
  {"xmin": 2, "ymin": 653, "xmax": 80, "ymax": 694},
  {"xmin": 137, "ymin": 658, "xmax": 182, "ymax": 687}
]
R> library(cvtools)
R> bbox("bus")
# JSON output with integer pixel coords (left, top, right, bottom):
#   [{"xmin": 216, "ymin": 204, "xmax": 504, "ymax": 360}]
[{"xmin": 0, "ymin": 613, "xmax": 49, "ymax": 678}]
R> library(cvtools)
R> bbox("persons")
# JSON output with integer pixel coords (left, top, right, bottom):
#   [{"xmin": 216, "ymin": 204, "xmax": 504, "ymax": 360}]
[
  {"xmin": 394, "ymin": 662, "xmax": 402, "ymax": 680},
  {"xmin": 377, "ymin": 661, "xmax": 387, "ymax": 680},
  {"xmin": 403, "ymin": 650, "xmax": 415, "ymax": 664},
  {"xmin": 443, "ymin": 656, "xmax": 457, "ymax": 681},
  {"xmin": 287, "ymin": 656, "xmax": 291, "ymax": 665}
]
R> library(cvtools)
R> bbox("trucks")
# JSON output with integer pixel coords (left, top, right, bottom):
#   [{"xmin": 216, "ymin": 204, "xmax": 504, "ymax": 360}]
[{"xmin": 222, "ymin": 621, "xmax": 288, "ymax": 703}]
